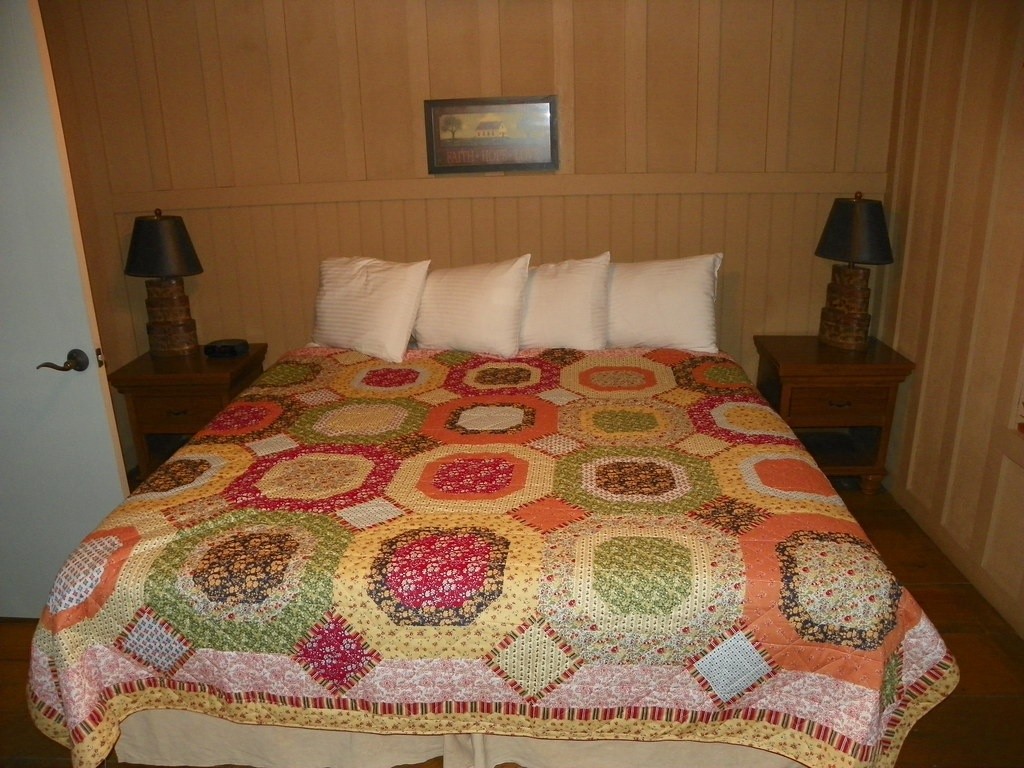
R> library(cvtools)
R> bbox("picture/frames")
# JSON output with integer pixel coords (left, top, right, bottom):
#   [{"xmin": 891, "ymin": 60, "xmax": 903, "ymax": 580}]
[{"xmin": 423, "ymin": 94, "xmax": 559, "ymax": 175}]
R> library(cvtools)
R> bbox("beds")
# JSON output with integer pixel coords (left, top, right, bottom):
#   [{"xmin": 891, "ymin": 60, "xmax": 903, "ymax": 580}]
[{"xmin": 27, "ymin": 173, "xmax": 964, "ymax": 768}]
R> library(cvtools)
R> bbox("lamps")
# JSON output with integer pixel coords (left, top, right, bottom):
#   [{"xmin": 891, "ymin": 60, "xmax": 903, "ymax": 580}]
[
  {"xmin": 122, "ymin": 208, "xmax": 204, "ymax": 361},
  {"xmin": 815, "ymin": 192, "xmax": 894, "ymax": 352}
]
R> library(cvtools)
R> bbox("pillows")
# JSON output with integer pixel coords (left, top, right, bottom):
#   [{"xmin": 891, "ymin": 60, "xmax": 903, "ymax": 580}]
[
  {"xmin": 525, "ymin": 251, "xmax": 619, "ymax": 358},
  {"xmin": 410, "ymin": 254, "xmax": 532, "ymax": 360},
  {"xmin": 305, "ymin": 257, "xmax": 431, "ymax": 365},
  {"xmin": 608, "ymin": 252, "xmax": 723, "ymax": 358}
]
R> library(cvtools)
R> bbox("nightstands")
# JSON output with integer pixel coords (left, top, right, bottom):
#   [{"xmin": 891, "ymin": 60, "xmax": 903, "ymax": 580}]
[
  {"xmin": 752, "ymin": 334, "xmax": 917, "ymax": 494},
  {"xmin": 107, "ymin": 342, "xmax": 269, "ymax": 482}
]
